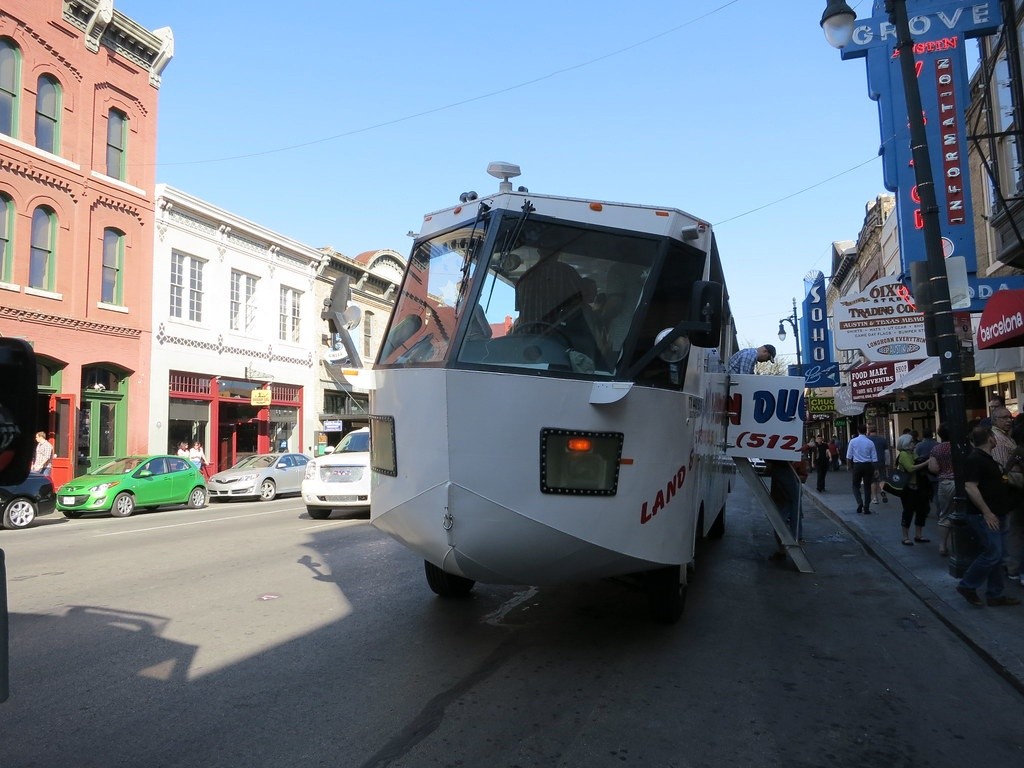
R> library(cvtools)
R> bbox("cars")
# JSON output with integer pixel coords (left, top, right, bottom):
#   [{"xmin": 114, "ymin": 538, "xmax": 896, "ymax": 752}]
[
  {"xmin": 748, "ymin": 458, "xmax": 770, "ymax": 474},
  {"xmin": 0, "ymin": 472, "xmax": 58, "ymax": 531},
  {"xmin": 56, "ymin": 454, "xmax": 209, "ymax": 520},
  {"xmin": 206, "ymin": 452, "xmax": 313, "ymax": 502}
]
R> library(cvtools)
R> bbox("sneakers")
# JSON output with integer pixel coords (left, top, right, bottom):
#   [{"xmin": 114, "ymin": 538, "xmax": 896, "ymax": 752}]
[
  {"xmin": 880, "ymin": 490, "xmax": 888, "ymax": 504},
  {"xmin": 871, "ymin": 497, "xmax": 879, "ymax": 504}
]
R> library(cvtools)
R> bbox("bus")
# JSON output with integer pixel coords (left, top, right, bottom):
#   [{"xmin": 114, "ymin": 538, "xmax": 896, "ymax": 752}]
[{"xmin": 324, "ymin": 158, "xmax": 819, "ymax": 624}]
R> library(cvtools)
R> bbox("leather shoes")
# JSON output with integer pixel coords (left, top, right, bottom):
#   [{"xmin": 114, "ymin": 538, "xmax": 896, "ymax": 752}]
[
  {"xmin": 980, "ymin": 593, "xmax": 1021, "ymax": 607},
  {"xmin": 955, "ymin": 584, "xmax": 984, "ymax": 609}
]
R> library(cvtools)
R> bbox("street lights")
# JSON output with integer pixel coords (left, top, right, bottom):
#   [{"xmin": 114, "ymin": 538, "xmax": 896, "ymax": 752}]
[{"xmin": 779, "ymin": 297, "xmax": 802, "ymax": 376}]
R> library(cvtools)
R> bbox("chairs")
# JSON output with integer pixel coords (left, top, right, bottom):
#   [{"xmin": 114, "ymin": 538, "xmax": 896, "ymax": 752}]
[
  {"xmin": 281, "ymin": 458, "xmax": 291, "ymax": 467},
  {"xmin": 171, "ymin": 462, "xmax": 185, "ymax": 472},
  {"xmin": 296, "ymin": 460, "xmax": 306, "ymax": 465},
  {"xmin": 148, "ymin": 461, "xmax": 164, "ymax": 476}
]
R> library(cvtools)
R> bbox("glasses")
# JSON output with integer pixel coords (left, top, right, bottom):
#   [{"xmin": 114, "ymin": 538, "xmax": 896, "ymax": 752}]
[{"xmin": 996, "ymin": 415, "xmax": 1016, "ymax": 422}]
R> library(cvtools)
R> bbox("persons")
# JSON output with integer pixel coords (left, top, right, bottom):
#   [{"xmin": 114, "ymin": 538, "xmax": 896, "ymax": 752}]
[
  {"xmin": 864, "ymin": 428, "xmax": 891, "ymax": 503},
  {"xmin": 845, "ymin": 426, "xmax": 880, "ymax": 512},
  {"xmin": 733, "ymin": 344, "xmax": 778, "ymax": 374},
  {"xmin": 904, "ymin": 427, "xmax": 922, "ymax": 447},
  {"xmin": 515, "ymin": 241, "xmax": 639, "ymax": 375},
  {"xmin": 176, "ymin": 441, "xmax": 208, "ymax": 473},
  {"xmin": 912, "ymin": 428, "xmax": 942, "ymax": 517},
  {"xmin": 957, "ymin": 426, "xmax": 1024, "ymax": 606},
  {"xmin": 928, "ymin": 422, "xmax": 963, "ymax": 556},
  {"xmin": 31, "ymin": 431, "xmax": 53, "ymax": 503},
  {"xmin": 458, "ymin": 278, "xmax": 492, "ymax": 357},
  {"xmin": 894, "ymin": 434, "xmax": 930, "ymax": 545},
  {"xmin": 984, "ymin": 398, "xmax": 1024, "ymax": 586},
  {"xmin": 809, "ymin": 435, "xmax": 842, "ymax": 492}
]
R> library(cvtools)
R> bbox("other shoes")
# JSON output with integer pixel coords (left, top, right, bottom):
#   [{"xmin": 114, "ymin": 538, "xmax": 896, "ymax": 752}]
[
  {"xmin": 823, "ymin": 487, "xmax": 825, "ymax": 491},
  {"xmin": 863, "ymin": 506, "xmax": 871, "ymax": 515},
  {"xmin": 856, "ymin": 503, "xmax": 863, "ymax": 513},
  {"xmin": 816, "ymin": 486, "xmax": 822, "ymax": 492},
  {"xmin": 901, "ymin": 538, "xmax": 914, "ymax": 546},
  {"xmin": 939, "ymin": 543, "xmax": 947, "ymax": 554},
  {"xmin": 915, "ymin": 535, "xmax": 931, "ymax": 543}
]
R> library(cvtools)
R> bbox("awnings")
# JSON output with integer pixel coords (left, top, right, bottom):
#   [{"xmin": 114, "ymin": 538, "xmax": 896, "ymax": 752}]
[{"xmin": 878, "ymin": 288, "xmax": 1023, "ymax": 397}]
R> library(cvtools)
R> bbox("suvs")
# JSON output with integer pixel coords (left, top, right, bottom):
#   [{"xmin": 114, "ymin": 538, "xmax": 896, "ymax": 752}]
[{"xmin": 300, "ymin": 426, "xmax": 371, "ymax": 519}]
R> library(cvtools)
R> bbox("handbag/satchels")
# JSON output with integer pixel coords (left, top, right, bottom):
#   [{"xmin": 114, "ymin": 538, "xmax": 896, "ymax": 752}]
[{"xmin": 883, "ymin": 467, "xmax": 911, "ymax": 498}]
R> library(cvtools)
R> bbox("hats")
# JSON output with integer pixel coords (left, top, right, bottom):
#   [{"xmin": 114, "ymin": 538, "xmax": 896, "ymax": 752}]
[{"xmin": 764, "ymin": 344, "xmax": 776, "ymax": 364}]
